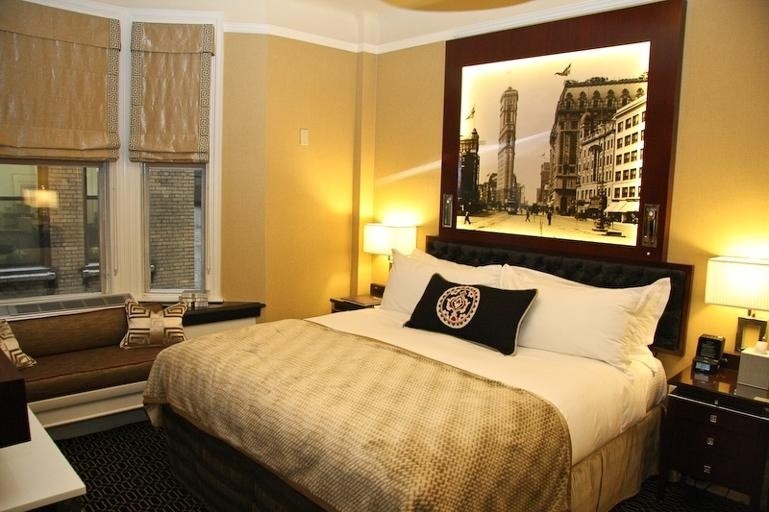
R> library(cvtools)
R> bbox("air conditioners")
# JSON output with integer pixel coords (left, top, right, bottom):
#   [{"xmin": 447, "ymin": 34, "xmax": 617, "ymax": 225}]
[{"xmin": 0, "ymin": 292, "xmax": 135, "ymax": 319}]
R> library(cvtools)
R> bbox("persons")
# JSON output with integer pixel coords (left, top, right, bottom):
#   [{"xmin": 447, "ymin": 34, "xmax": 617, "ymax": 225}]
[
  {"xmin": 547, "ymin": 209, "xmax": 553, "ymax": 225},
  {"xmin": 464, "ymin": 210, "xmax": 471, "ymax": 225},
  {"xmin": 525, "ymin": 209, "xmax": 530, "ymax": 222}
]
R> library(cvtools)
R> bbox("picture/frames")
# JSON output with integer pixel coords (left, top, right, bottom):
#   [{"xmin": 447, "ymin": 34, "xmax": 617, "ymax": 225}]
[{"xmin": 438, "ymin": 0, "xmax": 688, "ymax": 265}]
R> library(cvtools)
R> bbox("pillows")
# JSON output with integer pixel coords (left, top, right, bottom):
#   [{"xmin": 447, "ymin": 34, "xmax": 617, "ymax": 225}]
[
  {"xmin": 0, "ymin": 316, "xmax": 39, "ymax": 371},
  {"xmin": 373, "ymin": 245, "xmax": 503, "ymax": 326},
  {"xmin": 402, "ymin": 272, "xmax": 538, "ymax": 357},
  {"xmin": 497, "ymin": 263, "xmax": 672, "ymax": 382},
  {"xmin": 118, "ymin": 293, "xmax": 192, "ymax": 351}
]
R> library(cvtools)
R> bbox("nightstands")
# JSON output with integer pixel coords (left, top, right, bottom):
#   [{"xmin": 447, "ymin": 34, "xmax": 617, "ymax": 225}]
[
  {"xmin": 329, "ymin": 293, "xmax": 382, "ymax": 313},
  {"xmin": 656, "ymin": 365, "xmax": 769, "ymax": 512}
]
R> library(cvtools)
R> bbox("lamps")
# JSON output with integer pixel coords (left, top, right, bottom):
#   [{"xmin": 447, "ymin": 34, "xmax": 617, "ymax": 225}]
[
  {"xmin": 21, "ymin": 184, "xmax": 61, "ymax": 226},
  {"xmin": 362, "ymin": 221, "xmax": 417, "ymax": 298},
  {"xmin": 704, "ymin": 255, "xmax": 769, "ymax": 371}
]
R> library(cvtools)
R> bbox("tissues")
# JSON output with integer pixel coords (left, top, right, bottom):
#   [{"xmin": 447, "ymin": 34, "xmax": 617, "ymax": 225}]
[{"xmin": 737, "ymin": 342, "xmax": 769, "ymax": 390}]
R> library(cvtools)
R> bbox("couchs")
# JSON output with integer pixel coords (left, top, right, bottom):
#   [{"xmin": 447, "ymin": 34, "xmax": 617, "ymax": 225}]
[{"xmin": 0, "ymin": 303, "xmax": 193, "ymax": 449}]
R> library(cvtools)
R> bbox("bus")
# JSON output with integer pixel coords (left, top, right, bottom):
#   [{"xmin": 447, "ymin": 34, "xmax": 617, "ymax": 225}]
[{"xmin": 507, "ymin": 199, "xmax": 518, "ymax": 215}]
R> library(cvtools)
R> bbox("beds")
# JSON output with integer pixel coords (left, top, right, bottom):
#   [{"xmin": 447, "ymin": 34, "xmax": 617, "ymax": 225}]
[{"xmin": 142, "ymin": 234, "xmax": 696, "ymax": 512}]
